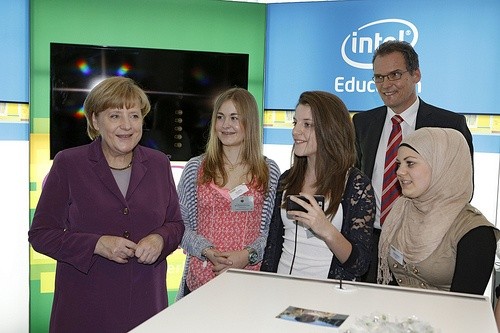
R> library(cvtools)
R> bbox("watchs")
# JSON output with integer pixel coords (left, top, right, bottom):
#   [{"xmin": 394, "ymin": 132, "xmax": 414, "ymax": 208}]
[{"xmin": 243, "ymin": 247, "xmax": 258, "ymax": 265}]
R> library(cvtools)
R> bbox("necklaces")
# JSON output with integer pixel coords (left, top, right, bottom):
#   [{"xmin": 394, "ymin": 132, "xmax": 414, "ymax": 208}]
[{"xmin": 108, "ymin": 161, "xmax": 132, "ymax": 170}]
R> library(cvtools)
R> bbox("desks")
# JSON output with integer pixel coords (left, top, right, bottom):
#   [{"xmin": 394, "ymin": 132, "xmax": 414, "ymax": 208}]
[{"xmin": 126, "ymin": 267, "xmax": 500, "ymax": 333}]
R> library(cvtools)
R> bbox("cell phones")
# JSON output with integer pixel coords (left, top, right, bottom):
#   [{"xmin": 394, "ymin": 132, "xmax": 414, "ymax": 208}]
[{"xmin": 285, "ymin": 195, "xmax": 325, "ymax": 214}]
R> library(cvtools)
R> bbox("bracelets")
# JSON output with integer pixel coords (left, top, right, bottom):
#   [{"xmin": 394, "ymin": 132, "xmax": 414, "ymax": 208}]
[{"xmin": 203, "ymin": 246, "xmax": 214, "ymax": 257}]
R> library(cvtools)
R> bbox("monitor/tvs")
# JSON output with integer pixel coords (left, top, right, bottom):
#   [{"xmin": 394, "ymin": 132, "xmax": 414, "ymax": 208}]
[{"xmin": 49, "ymin": 42, "xmax": 250, "ymax": 161}]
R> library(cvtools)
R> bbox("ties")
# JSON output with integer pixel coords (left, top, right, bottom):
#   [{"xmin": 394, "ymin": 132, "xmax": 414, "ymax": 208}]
[{"xmin": 379, "ymin": 114, "xmax": 404, "ymax": 228}]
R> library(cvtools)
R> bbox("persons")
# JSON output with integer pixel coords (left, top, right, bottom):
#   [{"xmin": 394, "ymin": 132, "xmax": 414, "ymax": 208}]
[
  {"xmin": 174, "ymin": 88, "xmax": 281, "ymax": 301},
  {"xmin": 28, "ymin": 78, "xmax": 186, "ymax": 333},
  {"xmin": 260, "ymin": 90, "xmax": 376, "ymax": 282},
  {"xmin": 353, "ymin": 41, "xmax": 473, "ymax": 286},
  {"xmin": 378, "ymin": 127, "xmax": 500, "ymax": 296}
]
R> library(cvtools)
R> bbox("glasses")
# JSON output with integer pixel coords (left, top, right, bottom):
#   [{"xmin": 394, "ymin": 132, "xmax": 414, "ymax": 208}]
[{"xmin": 371, "ymin": 71, "xmax": 407, "ymax": 83}]
